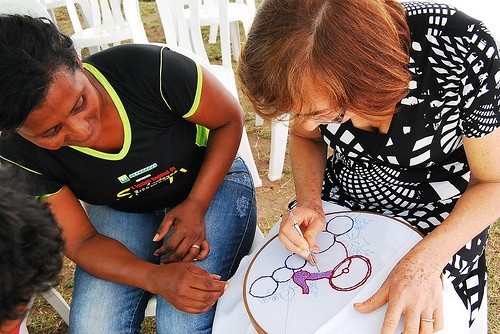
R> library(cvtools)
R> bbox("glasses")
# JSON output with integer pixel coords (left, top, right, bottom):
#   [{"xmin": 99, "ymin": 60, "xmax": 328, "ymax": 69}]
[{"xmin": 279, "ymin": 107, "xmax": 346, "ymax": 127}]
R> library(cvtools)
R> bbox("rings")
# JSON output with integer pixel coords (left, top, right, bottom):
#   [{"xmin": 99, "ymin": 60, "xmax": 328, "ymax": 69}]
[
  {"xmin": 421, "ymin": 318, "xmax": 435, "ymax": 322},
  {"xmin": 192, "ymin": 245, "xmax": 200, "ymax": 249}
]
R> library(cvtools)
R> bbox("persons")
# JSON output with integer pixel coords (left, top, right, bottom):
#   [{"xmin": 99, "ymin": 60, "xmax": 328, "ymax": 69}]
[
  {"xmin": 235, "ymin": 0, "xmax": 500, "ymax": 334},
  {"xmin": 0, "ymin": 160, "xmax": 66, "ymax": 334},
  {"xmin": 0, "ymin": 13, "xmax": 257, "ymax": 334}
]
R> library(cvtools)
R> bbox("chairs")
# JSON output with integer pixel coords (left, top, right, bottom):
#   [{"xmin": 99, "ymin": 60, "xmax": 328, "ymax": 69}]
[{"xmin": 0, "ymin": 0, "xmax": 290, "ymax": 187}]
